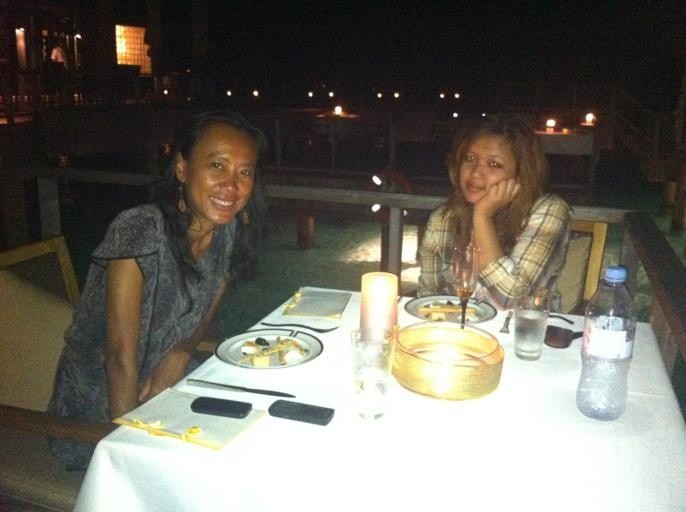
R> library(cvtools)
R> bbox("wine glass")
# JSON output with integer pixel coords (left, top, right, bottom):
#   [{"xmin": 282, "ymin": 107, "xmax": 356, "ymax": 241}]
[{"xmin": 453, "ymin": 246, "xmax": 482, "ymax": 326}]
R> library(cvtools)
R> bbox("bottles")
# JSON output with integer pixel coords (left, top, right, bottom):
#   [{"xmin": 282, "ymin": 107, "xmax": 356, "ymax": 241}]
[{"xmin": 575, "ymin": 267, "xmax": 640, "ymax": 422}]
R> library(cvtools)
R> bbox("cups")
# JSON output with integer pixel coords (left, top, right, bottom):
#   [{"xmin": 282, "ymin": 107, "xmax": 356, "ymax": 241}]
[
  {"xmin": 511, "ymin": 286, "xmax": 552, "ymax": 360},
  {"xmin": 351, "ymin": 326, "xmax": 392, "ymax": 418}
]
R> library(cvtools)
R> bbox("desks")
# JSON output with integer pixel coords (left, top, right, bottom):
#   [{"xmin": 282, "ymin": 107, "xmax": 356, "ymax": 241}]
[
  {"xmin": 275, "ymin": 106, "xmax": 396, "ymax": 189},
  {"xmin": 529, "ymin": 131, "xmax": 596, "ymax": 192},
  {"xmin": 73, "ymin": 285, "xmax": 686, "ymax": 512}
]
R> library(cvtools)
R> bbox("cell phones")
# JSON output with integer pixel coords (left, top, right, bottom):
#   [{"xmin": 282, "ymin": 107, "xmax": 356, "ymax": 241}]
[
  {"xmin": 191, "ymin": 396, "xmax": 253, "ymax": 419},
  {"xmin": 269, "ymin": 399, "xmax": 335, "ymax": 425}
]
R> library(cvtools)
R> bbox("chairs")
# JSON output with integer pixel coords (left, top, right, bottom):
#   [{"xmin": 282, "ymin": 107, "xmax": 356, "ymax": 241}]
[
  {"xmin": 1, "ymin": 231, "xmax": 219, "ymax": 512},
  {"xmin": 549, "ymin": 217, "xmax": 609, "ymax": 316}
]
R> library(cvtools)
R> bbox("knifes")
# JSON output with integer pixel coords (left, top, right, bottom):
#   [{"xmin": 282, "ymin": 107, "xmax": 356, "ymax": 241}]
[{"xmin": 187, "ymin": 377, "xmax": 296, "ymax": 401}]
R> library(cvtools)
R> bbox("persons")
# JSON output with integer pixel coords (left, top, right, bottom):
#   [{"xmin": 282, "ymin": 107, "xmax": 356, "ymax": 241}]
[
  {"xmin": 43, "ymin": 108, "xmax": 268, "ymax": 471},
  {"xmin": 407, "ymin": 109, "xmax": 575, "ymax": 318}
]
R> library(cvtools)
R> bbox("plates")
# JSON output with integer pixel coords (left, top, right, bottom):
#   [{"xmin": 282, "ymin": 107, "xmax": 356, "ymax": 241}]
[
  {"xmin": 214, "ymin": 328, "xmax": 323, "ymax": 369},
  {"xmin": 402, "ymin": 294, "xmax": 496, "ymax": 325}
]
